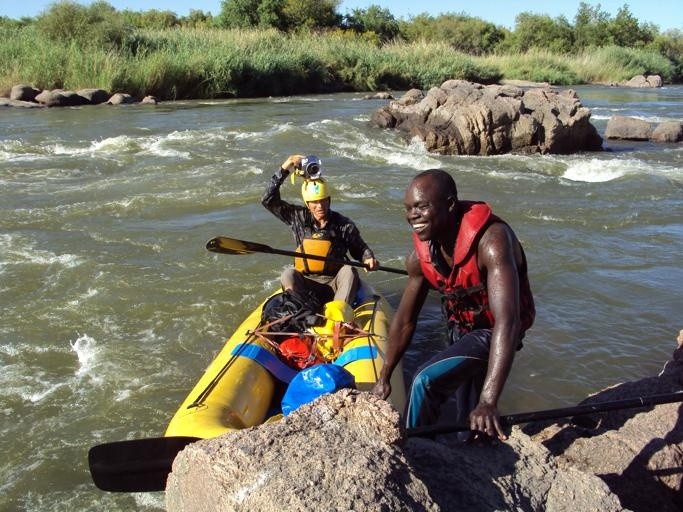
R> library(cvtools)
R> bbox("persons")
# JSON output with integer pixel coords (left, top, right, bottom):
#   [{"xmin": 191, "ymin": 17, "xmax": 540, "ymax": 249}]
[
  {"xmin": 259, "ymin": 155, "xmax": 380, "ymax": 305},
  {"xmin": 369, "ymin": 168, "xmax": 535, "ymax": 446}
]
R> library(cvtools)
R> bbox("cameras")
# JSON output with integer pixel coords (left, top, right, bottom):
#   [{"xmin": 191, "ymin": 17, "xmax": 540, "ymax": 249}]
[{"xmin": 294, "ymin": 154, "xmax": 323, "ymax": 180}]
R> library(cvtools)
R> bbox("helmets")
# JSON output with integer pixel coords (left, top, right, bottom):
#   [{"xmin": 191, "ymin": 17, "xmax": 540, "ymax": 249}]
[{"xmin": 301, "ymin": 179, "xmax": 332, "ymax": 208}]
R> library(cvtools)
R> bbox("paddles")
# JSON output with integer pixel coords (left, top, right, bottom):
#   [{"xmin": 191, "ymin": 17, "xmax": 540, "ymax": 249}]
[
  {"xmin": 207, "ymin": 236, "xmax": 408, "ymax": 275},
  {"xmin": 87, "ymin": 391, "xmax": 683, "ymax": 492}
]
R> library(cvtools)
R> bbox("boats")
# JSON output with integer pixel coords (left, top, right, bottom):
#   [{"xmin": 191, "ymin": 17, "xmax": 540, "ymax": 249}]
[{"xmin": 163, "ymin": 276, "xmax": 408, "ymax": 440}]
